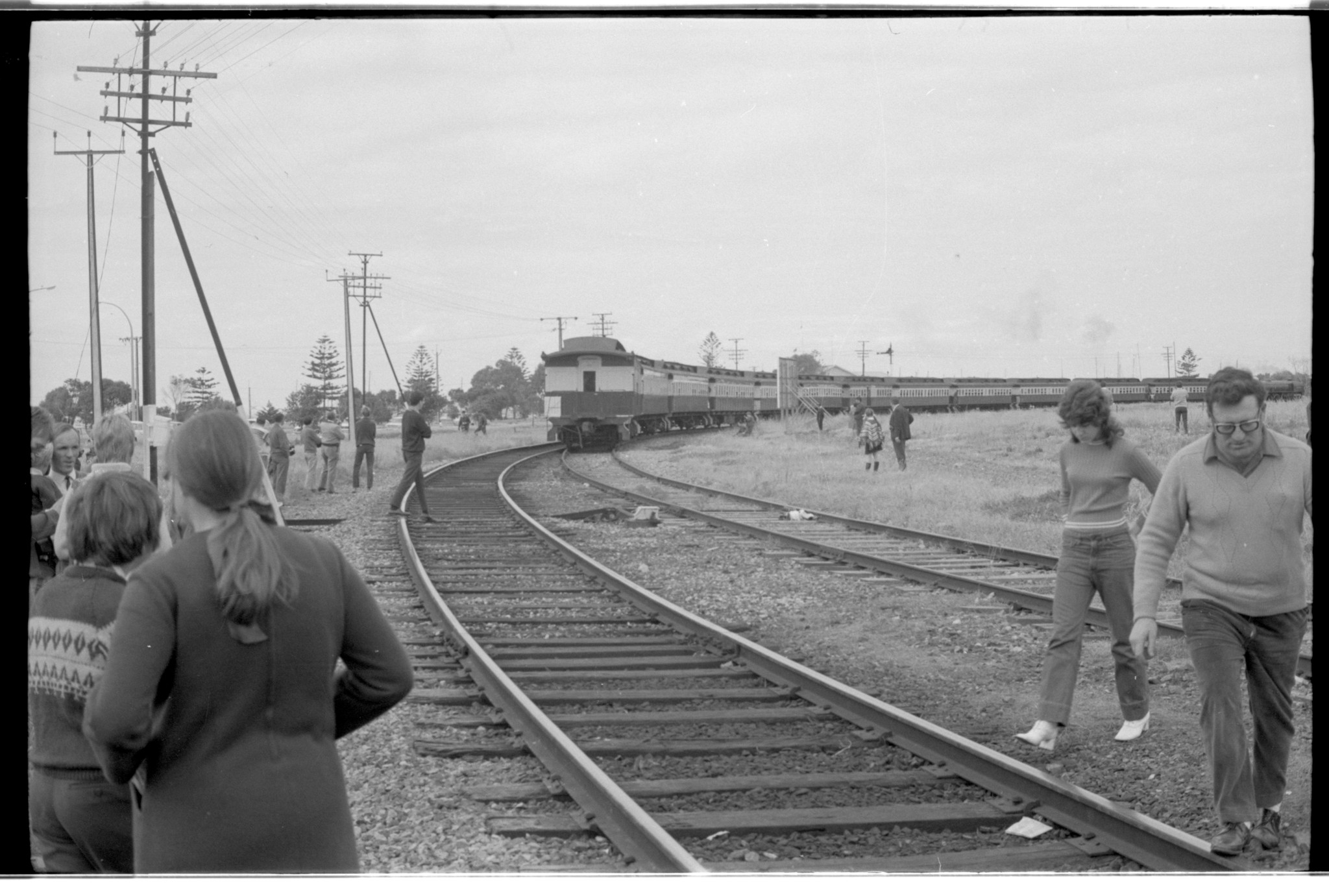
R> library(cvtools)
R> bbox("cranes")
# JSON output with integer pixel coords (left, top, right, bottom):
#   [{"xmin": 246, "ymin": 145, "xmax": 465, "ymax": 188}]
[{"xmin": 877, "ymin": 343, "xmax": 893, "ymax": 365}]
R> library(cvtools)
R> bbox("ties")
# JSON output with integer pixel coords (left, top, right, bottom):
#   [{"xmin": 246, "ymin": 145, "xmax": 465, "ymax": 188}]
[{"xmin": 64, "ymin": 475, "xmax": 71, "ymax": 491}]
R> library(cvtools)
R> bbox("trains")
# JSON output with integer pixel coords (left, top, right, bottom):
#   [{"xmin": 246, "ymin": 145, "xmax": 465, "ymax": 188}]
[{"xmin": 543, "ymin": 336, "xmax": 1304, "ymax": 455}]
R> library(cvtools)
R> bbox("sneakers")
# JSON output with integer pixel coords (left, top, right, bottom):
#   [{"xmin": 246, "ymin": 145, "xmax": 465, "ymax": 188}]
[
  {"xmin": 1250, "ymin": 808, "xmax": 1281, "ymax": 849},
  {"xmin": 1210, "ymin": 819, "xmax": 1256, "ymax": 857}
]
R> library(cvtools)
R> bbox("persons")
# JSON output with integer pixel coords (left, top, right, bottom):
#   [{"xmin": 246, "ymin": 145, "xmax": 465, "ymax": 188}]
[
  {"xmin": 848, "ymin": 397, "xmax": 865, "ymax": 440},
  {"xmin": 439, "ymin": 409, "xmax": 488, "ymax": 437},
  {"xmin": 859, "ymin": 408, "xmax": 885, "ymax": 471},
  {"xmin": 816, "ymin": 404, "xmax": 825, "ymax": 430},
  {"xmin": 28, "ymin": 404, "xmax": 173, "ymax": 874},
  {"xmin": 352, "ymin": 405, "xmax": 376, "ymax": 490},
  {"xmin": 1014, "ymin": 378, "xmax": 1162, "ymax": 751},
  {"xmin": 1130, "ymin": 367, "xmax": 1311, "ymax": 856},
  {"xmin": 80, "ymin": 411, "xmax": 415, "ymax": 873},
  {"xmin": 1169, "ymin": 381, "xmax": 1189, "ymax": 435},
  {"xmin": 889, "ymin": 396, "xmax": 914, "ymax": 471},
  {"xmin": 256, "ymin": 411, "xmax": 345, "ymax": 507},
  {"xmin": 1306, "ymin": 402, "xmax": 1312, "ymax": 446},
  {"xmin": 389, "ymin": 391, "xmax": 435, "ymax": 522},
  {"xmin": 734, "ymin": 411, "xmax": 755, "ymax": 437}
]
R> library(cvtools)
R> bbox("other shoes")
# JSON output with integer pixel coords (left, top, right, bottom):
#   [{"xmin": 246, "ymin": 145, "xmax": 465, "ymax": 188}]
[
  {"xmin": 385, "ymin": 508, "xmax": 412, "ymax": 518},
  {"xmin": 421, "ymin": 513, "xmax": 434, "ymax": 522}
]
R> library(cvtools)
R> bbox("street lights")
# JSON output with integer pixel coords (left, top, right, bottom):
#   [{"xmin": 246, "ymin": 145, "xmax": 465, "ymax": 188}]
[
  {"xmin": 119, "ymin": 336, "xmax": 144, "ymax": 422},
  {"xmin": 1164, "ymin": 347, "xmax": 1172, "ymax": 408},
  {"xmin": 98, "ymin": 302, "xmax": 137, "ymax": 421}
]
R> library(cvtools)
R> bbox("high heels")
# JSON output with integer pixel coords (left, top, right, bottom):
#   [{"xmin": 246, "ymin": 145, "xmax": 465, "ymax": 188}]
[
  {"xmin": 1114, "ymin": 709, "xmax": 1150, "ymax": 741},
  {"xmin": 1015, "ymin": 720, "xmax": 1058, "ymax": 751}
]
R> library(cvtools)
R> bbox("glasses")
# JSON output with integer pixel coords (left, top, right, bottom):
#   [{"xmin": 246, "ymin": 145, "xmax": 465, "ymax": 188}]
[{"xmin": 1211, "ymin": 407, "xmax": 1261, "ymax": 435}]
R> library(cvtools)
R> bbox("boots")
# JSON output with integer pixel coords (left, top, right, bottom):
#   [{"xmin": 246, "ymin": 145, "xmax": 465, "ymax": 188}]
[
  {"xmin": 873, "ymin": 461, "xmax": 879, "ymax": 472},
  {"xmin": 866, "ymin": 463, "xmax": 871, "ymax": 470}
]
